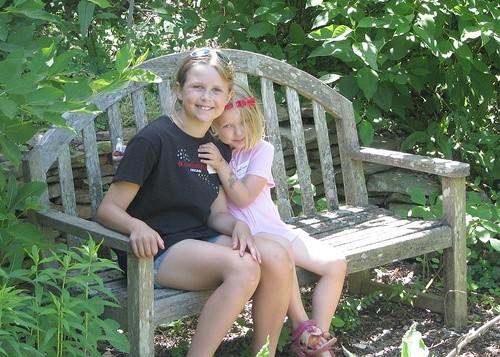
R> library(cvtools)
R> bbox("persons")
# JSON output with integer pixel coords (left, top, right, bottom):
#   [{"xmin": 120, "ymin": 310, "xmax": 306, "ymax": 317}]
[
  {"xmin": 95, "ymin": 47, "xmax": 292, "ymax": 356},
  {"xmin": 197, "ymin": 78, "xmax": 348, "ymax": 357}
]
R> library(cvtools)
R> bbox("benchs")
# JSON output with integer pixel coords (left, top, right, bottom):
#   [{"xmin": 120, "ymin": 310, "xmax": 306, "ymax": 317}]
[{"xmin": 22, "ymin": 49, "xmax": 470, "ymax": 356}]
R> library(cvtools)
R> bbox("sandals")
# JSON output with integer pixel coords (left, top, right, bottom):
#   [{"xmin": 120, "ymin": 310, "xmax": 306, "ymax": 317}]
[{"xmin": 290, "ymin": 319, "xmax": 337, "ymax": 357}]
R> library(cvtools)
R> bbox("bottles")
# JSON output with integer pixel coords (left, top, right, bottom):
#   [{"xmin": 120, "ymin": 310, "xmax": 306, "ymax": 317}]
[{"xmin": 114, "ymin": 138, "xmax": 126, "ymax": 170}]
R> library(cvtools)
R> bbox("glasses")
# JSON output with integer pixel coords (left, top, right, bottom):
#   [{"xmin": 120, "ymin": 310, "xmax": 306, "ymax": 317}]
[{"xmin": 189, "ymin": 47, "xmax": 232, "ymax": 65}]
